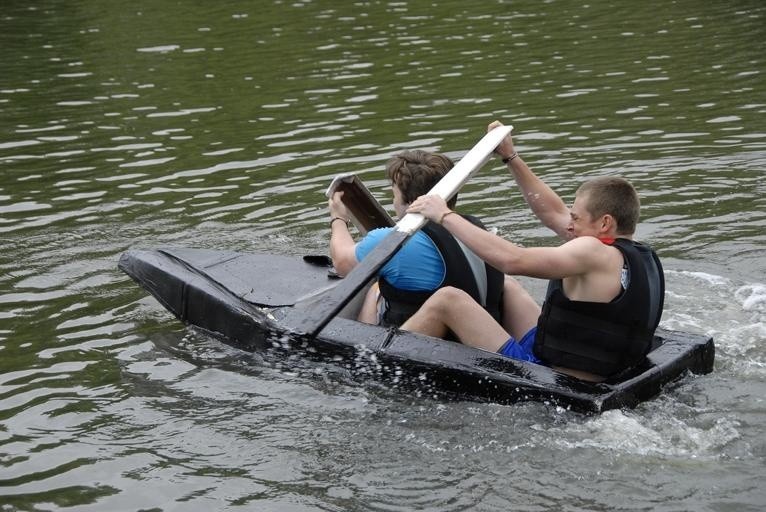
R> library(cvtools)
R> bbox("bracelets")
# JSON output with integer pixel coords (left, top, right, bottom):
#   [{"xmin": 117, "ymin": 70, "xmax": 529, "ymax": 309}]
[
  {"xmin": 502, "ymin": 151, "xmax": 520, "ymax": 164},
  {"xmin": 330, "ymin": 217, "xmax": 350, "ymax": 229}
]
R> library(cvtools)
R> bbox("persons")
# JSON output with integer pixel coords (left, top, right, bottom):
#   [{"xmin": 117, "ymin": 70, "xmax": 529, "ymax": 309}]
[
  {"xmin": 328, "ymin": 148, "xmax": 504, "ymax": 351},
  {"xmin": 395, "ymin": 119, "xmax": 665, "ymax": 381}
]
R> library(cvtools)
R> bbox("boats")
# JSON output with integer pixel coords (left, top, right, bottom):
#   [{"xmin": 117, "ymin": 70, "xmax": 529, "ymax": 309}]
[{"xmin": 116, "ymin": 247, "xmax": 717, "ymax": 427}]
